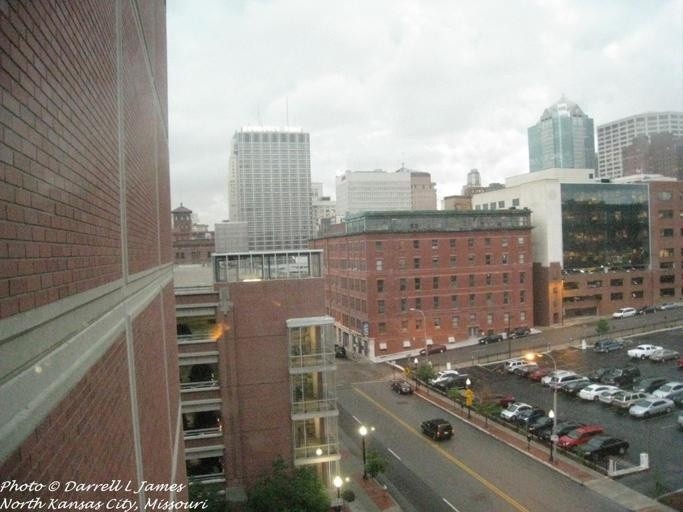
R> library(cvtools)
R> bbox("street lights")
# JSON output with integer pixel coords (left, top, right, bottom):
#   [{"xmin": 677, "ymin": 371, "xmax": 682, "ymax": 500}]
[
  {"xmin": 358, "ymin": 425, "xmax": 368, "ymax": 481},
  {"xmin": 409, "ymin": 308, "xmax": 428, "ymax": 361},
  {"xmin": 333, "ymin": 475, "xmax": 342, "ymax": 512},
  {"xmin": 525, "ymin": 351, "xmax": 557, "ymax": 464}
]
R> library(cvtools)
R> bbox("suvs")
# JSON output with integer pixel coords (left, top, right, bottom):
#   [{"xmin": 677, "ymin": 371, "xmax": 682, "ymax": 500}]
[
  {"xmin": 592, "ymin": 338, "xmax": 624, "ymax": 353},
  {"xmin": 507, "ymin": 325, "xmax": 532, "ymax": 339},
  {"xmin": 420, "ymin": 418, "xmax": 454, "ymax": 441}
]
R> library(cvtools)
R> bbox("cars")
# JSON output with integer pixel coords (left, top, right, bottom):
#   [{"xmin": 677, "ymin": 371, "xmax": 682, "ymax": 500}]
[
  {"xmin": 627, "ymin": 344, "xmax": 683, "ymax": 369},
  {"xmin": 504, "ymin": 359, "xmax": 592, "ymax": 395},
  {"xmin": 426, "ymin": 370, "xmax": 472, "ymax": 392},
  {"xmin": 391, "ymin": 381, "xmax": 413, "ymax": 395},
  {"xmin": 558, "ymin": 425, "xmax": 629, "ymax": 462},
  {"xmin": 612, "ymin": 302, "xmax": 679, "ymax": 319},
  {"xmin": 479, "ymin": 335, "xmax": 503, "ymax": 344},
  {"xmin": 420, "ymin": 344, "xmax": 447, "ymax": 356},
  {"xmin": 528, "ymin": 416, "xmax": 585, "ymax": 442},
  {"xmin": 577, "ymin": 367, "xmax": 683, "ymax": 418},
  {"xmin": 478, "ymin": 392, "xmax": 545, "ymax": 426}
]
